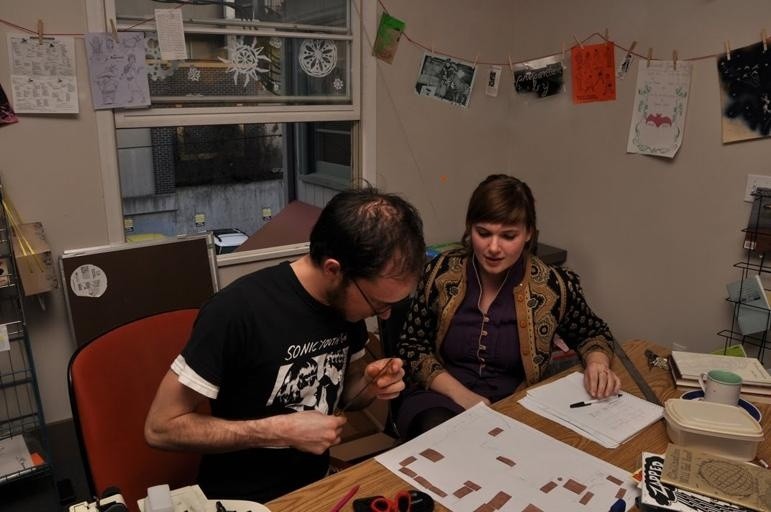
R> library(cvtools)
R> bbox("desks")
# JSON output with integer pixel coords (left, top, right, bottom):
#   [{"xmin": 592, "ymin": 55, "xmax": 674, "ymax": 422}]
[{"xmin": 265, "ymin": 338, "xmax": 771, "ymax": 510}]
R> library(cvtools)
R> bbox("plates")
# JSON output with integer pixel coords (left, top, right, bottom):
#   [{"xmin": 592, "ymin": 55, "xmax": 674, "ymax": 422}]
[{"xmin": 679, "ymin": 389, "xmax": 762, "ymax": 425}]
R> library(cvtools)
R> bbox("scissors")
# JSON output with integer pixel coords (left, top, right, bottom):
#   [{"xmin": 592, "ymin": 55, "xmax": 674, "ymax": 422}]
[{"xmin": 371, "ymin": 490, "xmax": 412, "ymax": 512}]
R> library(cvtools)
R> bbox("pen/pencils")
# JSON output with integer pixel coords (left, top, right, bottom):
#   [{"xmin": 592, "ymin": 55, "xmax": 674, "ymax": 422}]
[
  {"xmin": 326, "ymin": 485, "xmax": 360, "ymax": 512},
  {"xmin": 570, "ymin": 393, "xmax": 625, "ymax": 408},
  {"xmin": 216, "ymin": 500, "xmax": 228, "ymax": 512},
  {"xmin": 607, "ymin": 498, "xmax": 627, "ymax": 512}
]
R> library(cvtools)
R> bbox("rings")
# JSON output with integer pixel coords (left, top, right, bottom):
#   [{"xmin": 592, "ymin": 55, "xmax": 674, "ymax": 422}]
[{"xmin": 600, "ymin": 370, "xmax": 609, "ymax": 376}]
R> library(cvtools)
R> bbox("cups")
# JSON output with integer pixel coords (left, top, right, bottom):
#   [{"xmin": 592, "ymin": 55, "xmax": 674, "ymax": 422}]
[{"xmin": 699, "ymin": 369, "xmax": 743, "ymax": 406}]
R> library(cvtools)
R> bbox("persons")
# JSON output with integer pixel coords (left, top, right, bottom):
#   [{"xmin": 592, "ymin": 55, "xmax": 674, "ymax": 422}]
[
  {"xmin": 123, "ymin": 53, "xmax": 147, "ymax": 106},
  {"xmin": 388, "ymin": 173, "xmax": 623, "ymax": 441},
  {"xmin": 318, "ymin": 346, "xmax": 346, "ymax": 416},
  {"xmin": 270, "ymin": 358, "xmax": 321, "ymax": 414},
  {"xmin": 141, "ymin": 183, "xmax": 429, "ymax": 510}
]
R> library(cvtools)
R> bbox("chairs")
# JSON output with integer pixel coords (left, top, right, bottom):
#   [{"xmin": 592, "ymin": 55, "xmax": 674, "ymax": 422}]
[{"xmin": 65, "ymin": 305, "xmax": 213, "ymax": 505}]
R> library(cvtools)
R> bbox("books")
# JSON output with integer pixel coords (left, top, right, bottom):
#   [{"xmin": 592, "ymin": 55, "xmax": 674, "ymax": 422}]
[
  {"xmin": 742, "ymin": 186, "xmax": 771, "ymax": 261},
  {"xmin": 0, "ymin": 433, "xmax": 36, "ymax": 485},
  {"xmin": 669, "ymin": 343, "xmax": 771, "ymax": 404},
  {"xmin": 728, "ymin": 274, "xmax": 771, "ymax": 337},
  {"xmin": 629, "ymin": 439, "xmax": 771, "ymax": 512},
  {"xmin": 518, "ymin": 368, "xmax": 668, "ymax": 452}
]
